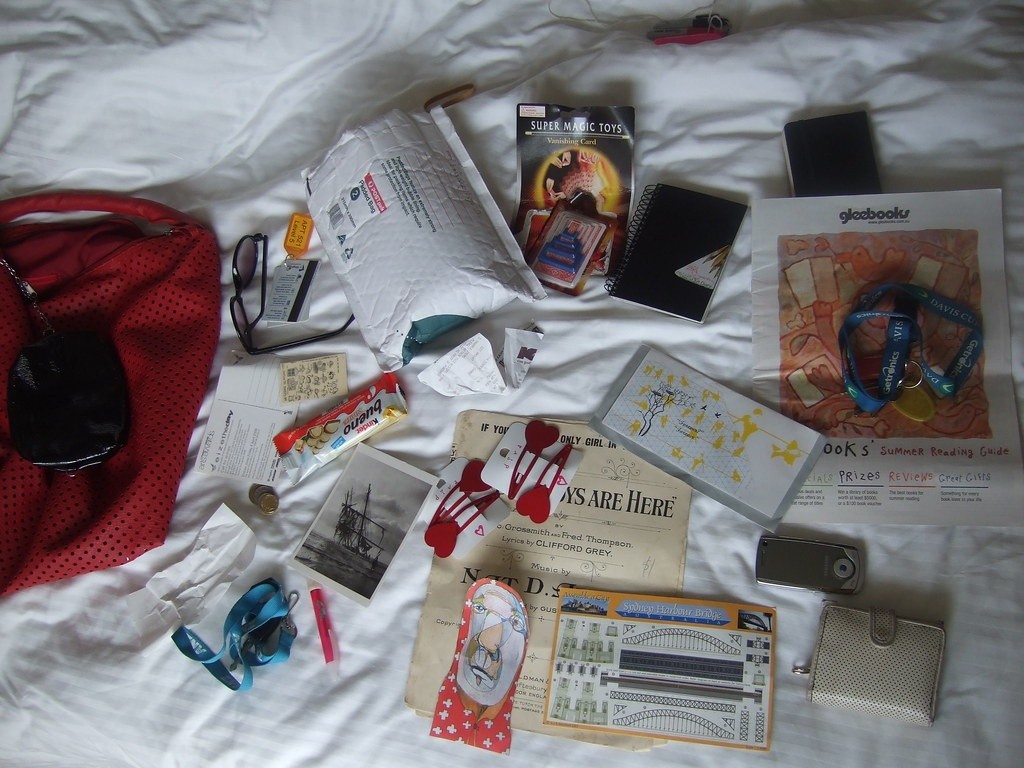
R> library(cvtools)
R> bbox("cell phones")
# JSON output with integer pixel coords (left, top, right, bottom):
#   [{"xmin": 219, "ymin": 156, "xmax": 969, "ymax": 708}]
[{"xmin": 755, "ymin": 536, "xmax": 863, "ymax": 595}]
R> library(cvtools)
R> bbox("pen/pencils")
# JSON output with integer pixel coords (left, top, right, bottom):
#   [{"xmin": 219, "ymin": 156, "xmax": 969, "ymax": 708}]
[{"xmin": 304, "ymin": 576, "xmax": 342, "ymax": 680}]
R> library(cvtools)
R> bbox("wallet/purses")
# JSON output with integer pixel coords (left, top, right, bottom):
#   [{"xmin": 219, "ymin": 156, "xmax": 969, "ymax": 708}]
[{"xmin": 793, "ymin": 598, "xmax": 946, "ymax": 725}]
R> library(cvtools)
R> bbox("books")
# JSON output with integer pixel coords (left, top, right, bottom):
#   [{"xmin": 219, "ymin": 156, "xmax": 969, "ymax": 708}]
[
  {"xmin": 603, "ymin": 179, "xmax": 750, "ymax": 323},
  {"xmin": 782, "ymin": 110, "xmax": 881, "ymax": 198}
]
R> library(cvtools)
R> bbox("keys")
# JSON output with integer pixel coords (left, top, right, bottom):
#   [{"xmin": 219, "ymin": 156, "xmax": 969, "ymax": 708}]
[{"xmin": 840, "ymin": 368, "xmax": 905, "ymax": 398}]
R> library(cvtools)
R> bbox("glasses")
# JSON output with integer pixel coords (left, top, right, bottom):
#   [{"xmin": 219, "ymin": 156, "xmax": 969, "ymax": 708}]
[{"xmin": 229, "ymin": 232, "xmax": 355, "ymax": 356}]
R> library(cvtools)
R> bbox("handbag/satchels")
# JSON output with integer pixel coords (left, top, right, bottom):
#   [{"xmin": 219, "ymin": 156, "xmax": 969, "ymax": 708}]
[
  {"xmin": 0, "ymin": 189, "xmax": 223, "ymax": 595},
  {"xmin": 4, "ymin": 328, "xmax": 133, "ymax": 475}
]
